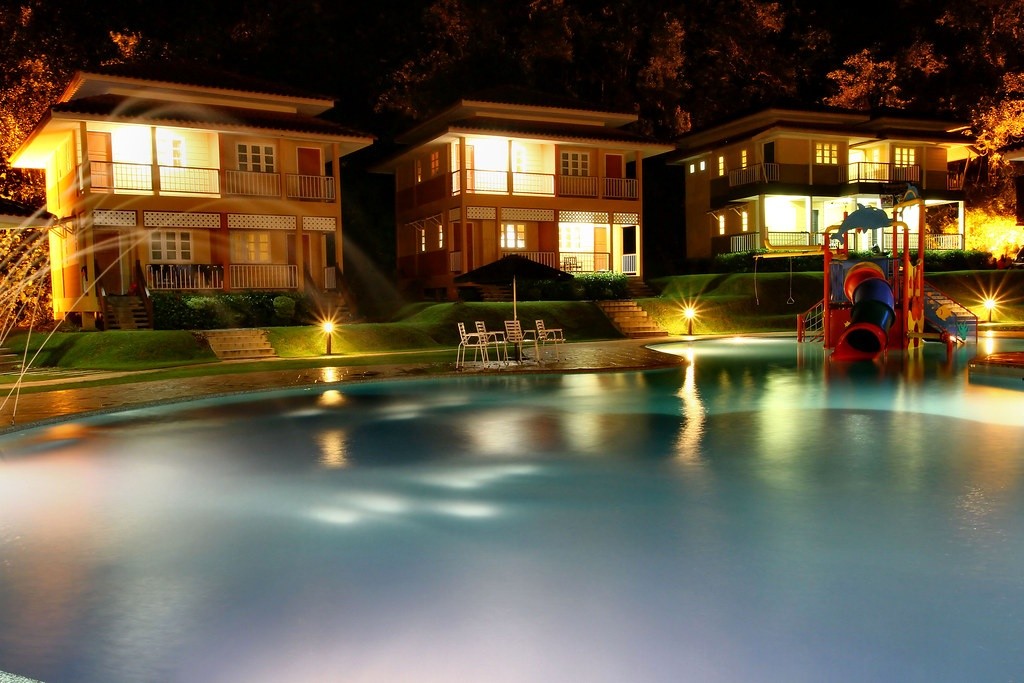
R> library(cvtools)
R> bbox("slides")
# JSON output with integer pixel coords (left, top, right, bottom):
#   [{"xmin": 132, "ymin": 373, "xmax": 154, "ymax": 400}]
[{"xmin": 833, "ymin": 260, "xmax": 895, "ymax": 362}]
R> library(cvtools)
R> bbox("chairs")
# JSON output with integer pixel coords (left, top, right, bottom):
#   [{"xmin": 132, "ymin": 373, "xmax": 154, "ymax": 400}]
[
  {"xmin": 474, "ymin": 321, "xmax": 509, "ymax": 371},
  {"xmin": 503, "ymin": 320, "xmax": 540, "ymax": 366},
  {"xmin": 456, "ymin": 322, "xmax": 490, "ymax": 369},
  {"xmin": 535, "ymin": 320, "xmax": 567, "ymax": 362}
]
